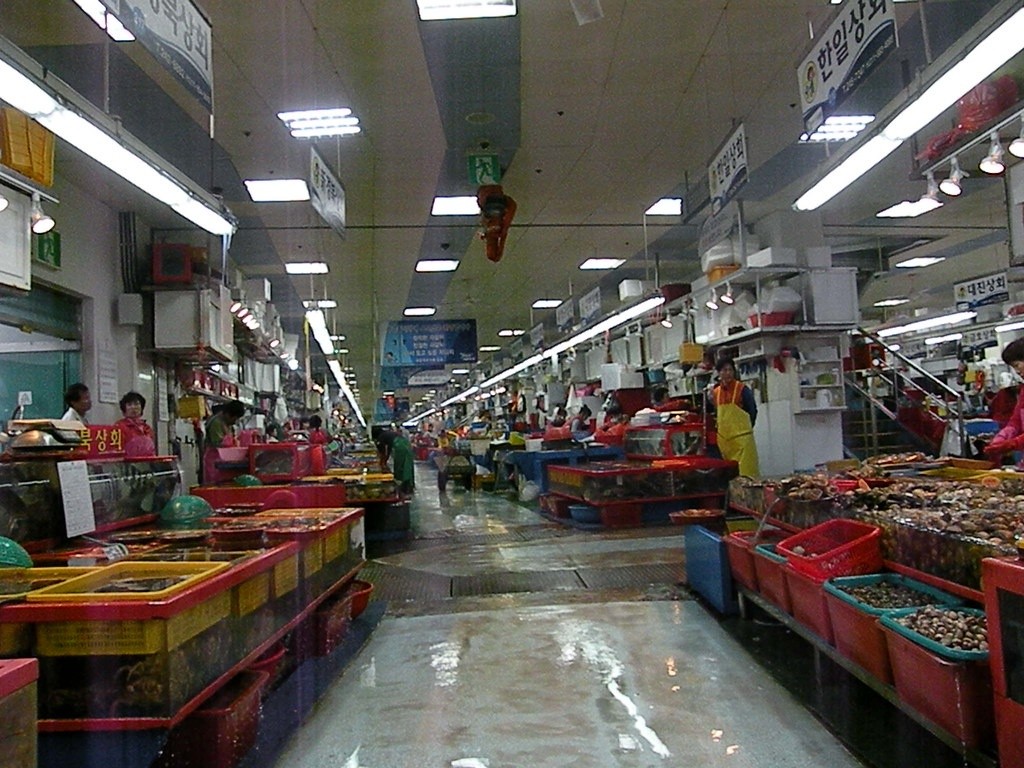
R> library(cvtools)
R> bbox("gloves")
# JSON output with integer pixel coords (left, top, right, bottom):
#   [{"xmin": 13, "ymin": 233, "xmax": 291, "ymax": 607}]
[
  {"xmin": 989, "ymin": 437, "xmax": 1008, "ymax": 467},
  {"xmin": 983, "ymin": 434, "xmax": 1024, "ymax": 454}
]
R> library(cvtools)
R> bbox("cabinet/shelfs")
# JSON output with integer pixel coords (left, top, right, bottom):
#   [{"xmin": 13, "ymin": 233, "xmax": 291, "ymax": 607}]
[
  {"xmin": 787, "ymin": 356, "xmax": 850, "ymax": 416},
  {"xmin": 153, "ymin": 288, "xmax": 235, "ymax": 363}
]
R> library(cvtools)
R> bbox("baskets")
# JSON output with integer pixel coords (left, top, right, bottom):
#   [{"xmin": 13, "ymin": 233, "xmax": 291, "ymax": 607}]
[{"xmin": 0, "ymin": 509, "xmax": 364, "ymax": 656}]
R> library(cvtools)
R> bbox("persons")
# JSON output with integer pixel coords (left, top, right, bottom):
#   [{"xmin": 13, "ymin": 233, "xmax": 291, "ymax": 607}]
[
  {"xmin": 595, "ymin": 406, "xmax": 626, "ymax": 444},
  {"xmin": 198, "ymin": 400, "xmax": 245, "ymax": 485},
  {"xmin": 654, "ymin": 387, "xmax": 690, "ymax": 409},
  {"xmin": 60, "ymin": 383, "xmax": 92, "ymax": 424},
  {"xmin": 113, "ymin": 391, "xmax": 154, "ymax": 450},
  {"xmin": 560, "ymin": 404, "xmax": 592, "ymax": 440},
  {"xmin": 266, "ymin": 415, "xmax": 415, "ymax": 499},
  {"xmin": 712, "ymin": 358, "xmax": 758, "ymax": 482},
  {"xmin": 422, "ymin": 423, "xmax": 437, "ymax": 444},
  {"xmin": 984, "ymin": 336, "xmax": 1024, "ymax": 468}
]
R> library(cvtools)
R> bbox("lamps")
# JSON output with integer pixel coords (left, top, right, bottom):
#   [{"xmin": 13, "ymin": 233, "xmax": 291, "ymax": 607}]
[
  {"xmin": 978, "ymin": 134, "xmax": 1005, "ymax": 175},
  {"xmin": 568, "ymin": 280, "xmax": 734, "ymax": 362},
  {"xmin": 31, "ymin": 194, "xmax": 55, "ymax": 233},
  {"xmin": 0, "ymin": 195, "xmax": 9, "ymax": 211},
  {"xmin": 1009, "ymin": 116, "xmax": 1024, "ymax": 159},
  {"xmin": 939, "ymin": 160, "xmax": 969, "ymax": 196}
]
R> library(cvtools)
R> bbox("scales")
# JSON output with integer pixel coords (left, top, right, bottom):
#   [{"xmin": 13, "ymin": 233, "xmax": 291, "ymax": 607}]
[{"xmin": 7, "ymin": 418, "xmax": 88, "ymax": 443}]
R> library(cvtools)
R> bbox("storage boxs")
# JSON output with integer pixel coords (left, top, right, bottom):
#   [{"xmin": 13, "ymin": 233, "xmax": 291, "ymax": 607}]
[{"xmin": 158, "ymin": 597, "xmax": 354, "ymax": 768}]
